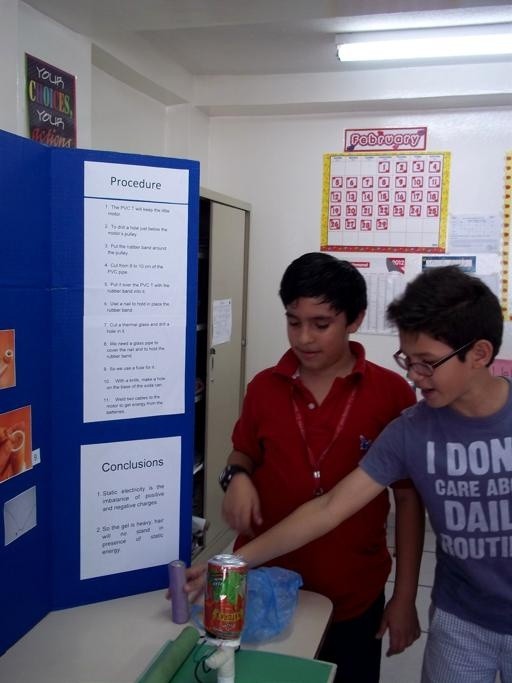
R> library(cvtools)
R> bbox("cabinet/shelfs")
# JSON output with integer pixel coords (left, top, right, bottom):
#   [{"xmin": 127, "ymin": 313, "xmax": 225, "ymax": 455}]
[{"xmin": 191, "ymin": 190, "xmax": 251, "ymax": 568}]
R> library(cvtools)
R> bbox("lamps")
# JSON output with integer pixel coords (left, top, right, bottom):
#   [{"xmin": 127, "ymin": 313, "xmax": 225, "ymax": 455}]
[{"xmin": 337, "ymin": 30, "xmax": 512, "ymax": 62}]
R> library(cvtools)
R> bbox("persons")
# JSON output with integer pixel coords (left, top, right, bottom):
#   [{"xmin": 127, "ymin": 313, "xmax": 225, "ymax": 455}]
[
  {"xmin": 218, "ymin": 251, "xmax": 427, "ymax": 682},
  {"xmin": 162, "ymin": 260, "xmax": 512, "ymax": 681}
]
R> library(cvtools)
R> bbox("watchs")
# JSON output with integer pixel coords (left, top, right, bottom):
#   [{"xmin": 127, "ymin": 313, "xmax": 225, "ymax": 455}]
[{"xmin": 218, "ymin": 463, "xmax": 249, "ymax": 492}]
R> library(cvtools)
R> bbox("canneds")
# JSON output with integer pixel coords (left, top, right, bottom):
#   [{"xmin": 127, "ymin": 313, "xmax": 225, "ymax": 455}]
[{"xmin": 204, "ymin": 554, "xmax": 249, "ymax": 639}]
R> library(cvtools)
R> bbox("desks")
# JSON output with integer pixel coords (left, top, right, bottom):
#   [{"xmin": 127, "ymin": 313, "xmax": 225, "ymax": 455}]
[{"xmin": 0, "ymin": 586, "xmax": 334, "ymax": 683}]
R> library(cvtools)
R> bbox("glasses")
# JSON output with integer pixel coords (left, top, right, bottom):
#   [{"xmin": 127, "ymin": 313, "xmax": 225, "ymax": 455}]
[{"xmin": 393, "ymin": 339, "xmax": 478, "ymax": 377}]
